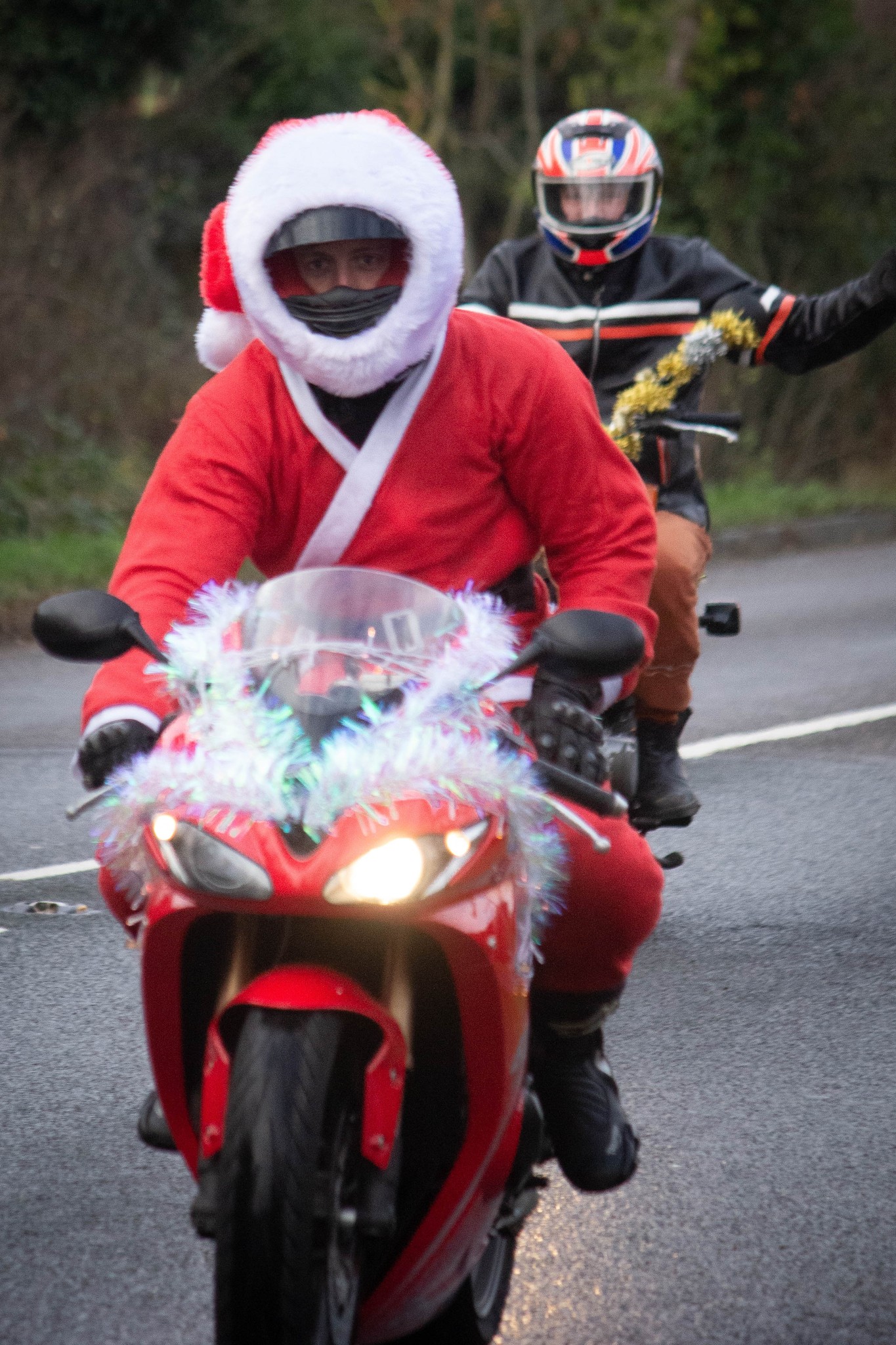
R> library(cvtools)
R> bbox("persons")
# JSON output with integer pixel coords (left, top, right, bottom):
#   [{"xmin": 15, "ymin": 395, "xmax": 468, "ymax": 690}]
[
  {"xmin": 77, "ymin": 107, "xmax": 670, "ymax": 1199},
  {"xmin": 446, "ymin": 109, "xmax": 894, "ymax": 829}
]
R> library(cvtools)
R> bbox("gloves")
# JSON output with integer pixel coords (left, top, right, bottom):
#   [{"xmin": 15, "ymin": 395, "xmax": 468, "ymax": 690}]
[
  {"xmin": 73, "ymin": 720, "xmax": 156, "ymax": 790},
  {"xmin": 511, "ymin": 661, "xmax": 611, "ymax": 793}
]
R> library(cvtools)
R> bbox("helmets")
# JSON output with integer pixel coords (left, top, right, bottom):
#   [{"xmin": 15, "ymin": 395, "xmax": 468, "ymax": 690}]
[
  {"xmin": 262, "ymin": 207, "xmax": 409, "ymax": 341},
  {"xmin": 530, "ymin": 108, "xmax": 662, "ymax": 266}
]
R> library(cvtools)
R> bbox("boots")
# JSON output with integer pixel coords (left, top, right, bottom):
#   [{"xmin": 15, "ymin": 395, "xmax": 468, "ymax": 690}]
[
  {"xmin": 524, "ymin": 990, "xmax": 640, "ymax": 1190},
  {"xmin": 137, "ymin": 1086, "xmax": 178, "ymax": 1156},
  {"xmin": 636, "ymin": 708, "xmax": 700, "ymax": 818}
]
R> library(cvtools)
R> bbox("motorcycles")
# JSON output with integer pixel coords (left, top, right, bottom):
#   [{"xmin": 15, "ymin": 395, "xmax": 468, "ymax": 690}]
[{"xmin": 34, "ymin": 584, "xmax": 684, "ymax": 1345}]
